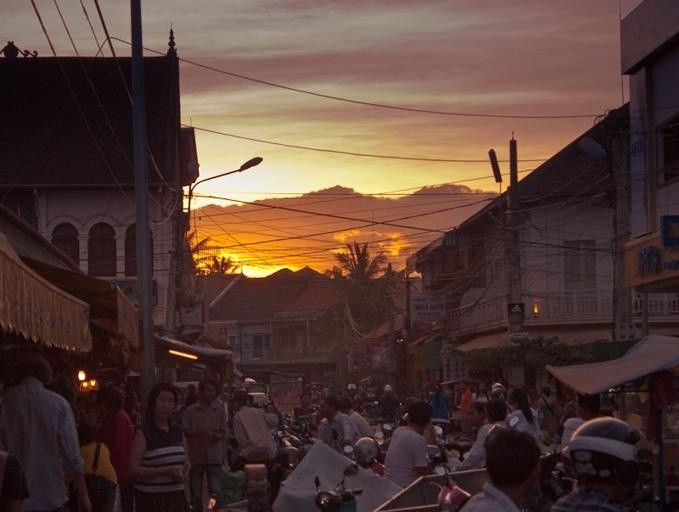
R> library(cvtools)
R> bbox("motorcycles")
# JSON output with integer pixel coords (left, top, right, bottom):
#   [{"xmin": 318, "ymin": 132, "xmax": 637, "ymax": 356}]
[
  {"xmin": 315, "ymin": 464, "xmax": 362, "ymax": 512},
  {"xmin": 429, "ymin": 426, "xmax": 472, "ymax": 512}
]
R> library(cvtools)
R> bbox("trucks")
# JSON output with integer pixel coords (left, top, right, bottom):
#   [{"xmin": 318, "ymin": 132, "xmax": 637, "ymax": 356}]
[{"xmin": 244, "ymin": 382, "xmax": 271, "ymax": 407}]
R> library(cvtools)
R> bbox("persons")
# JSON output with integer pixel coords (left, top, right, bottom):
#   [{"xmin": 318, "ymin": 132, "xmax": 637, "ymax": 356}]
[{"xmin": 1, "ymin": 356, "xmax": 652, "ymax": 511}]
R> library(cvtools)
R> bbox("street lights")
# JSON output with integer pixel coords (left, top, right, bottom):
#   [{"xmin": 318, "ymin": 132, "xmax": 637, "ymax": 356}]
[{"xmin": 189, "ymin": 157, "xmax": 263, "ymax": 211}]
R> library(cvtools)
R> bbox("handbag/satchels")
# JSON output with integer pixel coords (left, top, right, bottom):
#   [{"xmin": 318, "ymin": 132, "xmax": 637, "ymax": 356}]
[{"xmin": 69, "ymin": 472, "xmax": 118, "ymax": 511}]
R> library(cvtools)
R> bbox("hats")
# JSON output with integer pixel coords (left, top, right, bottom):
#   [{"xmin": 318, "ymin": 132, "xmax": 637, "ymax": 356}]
[{"xmin": 484, "ymin": 426, "xmax": 540, "ymax": 489}]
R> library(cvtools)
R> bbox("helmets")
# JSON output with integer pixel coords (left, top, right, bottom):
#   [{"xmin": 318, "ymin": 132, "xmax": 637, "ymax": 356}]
[
  {"xmin": 383, "ymin": 384, "xmax": 393, "ymax": 397},
  {"xmin": 492, "ymin": 382, "xmax": 505, "ymax": 392},
  {"xmin": 354, "ymin": 435, "xmax": 381, "ymax": 468},
  {"xmin": 569, "ymin": 416, "xmax": 648, "ymax": 487},
  {"xmin": 347, "ymin": 383, "xmax": 357, "ymax": 391}
]
